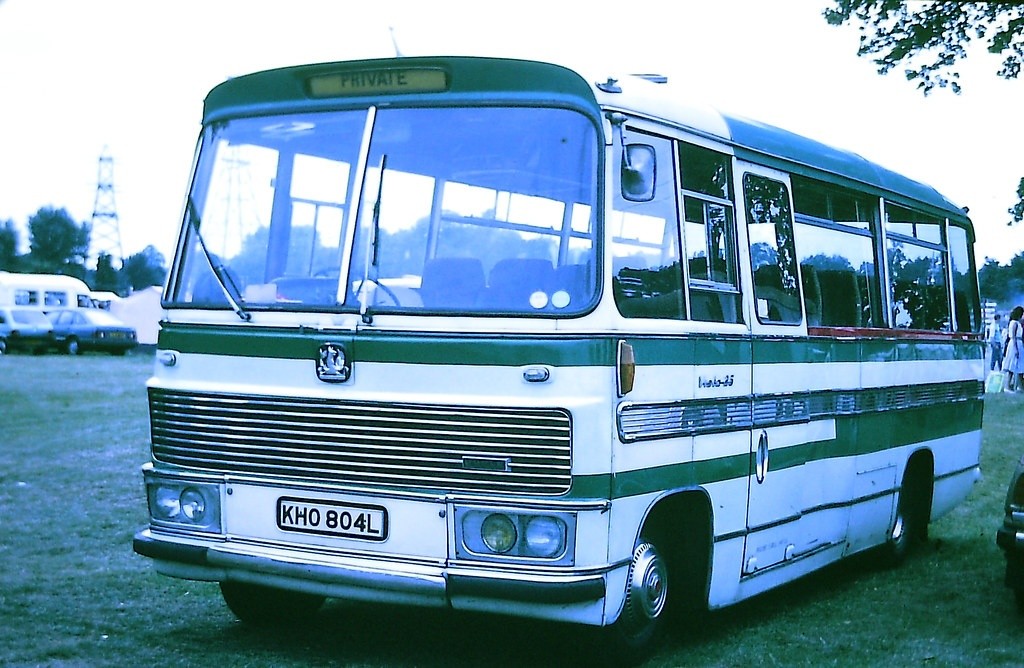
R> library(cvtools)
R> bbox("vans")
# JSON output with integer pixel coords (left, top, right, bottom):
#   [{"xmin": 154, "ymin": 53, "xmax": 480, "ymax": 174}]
[{"xmin": 0, "ymin": 271, "xmax": 99, "ymax": 313}]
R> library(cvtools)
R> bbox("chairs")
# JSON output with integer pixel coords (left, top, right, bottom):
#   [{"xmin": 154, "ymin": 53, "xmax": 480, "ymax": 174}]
[{"xmin": 423, "ymin": 258, "xmax": 972, "ymax": 332}]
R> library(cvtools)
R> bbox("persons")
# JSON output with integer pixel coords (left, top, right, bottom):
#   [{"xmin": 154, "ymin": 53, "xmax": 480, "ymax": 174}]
[{"xmin": 990, "ymin": 304, "xmax": 1024, "ymax": 394}]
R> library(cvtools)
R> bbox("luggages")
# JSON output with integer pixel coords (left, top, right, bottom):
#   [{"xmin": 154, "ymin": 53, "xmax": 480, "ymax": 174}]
[{"xmin": 985, "ymin": 371, "xmax": 1003, "ymax": 392}]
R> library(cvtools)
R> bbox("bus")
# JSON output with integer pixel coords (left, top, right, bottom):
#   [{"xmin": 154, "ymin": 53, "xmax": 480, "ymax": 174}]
[{"xmin": 133, "ymin": 51, "xmax": 988, "ymax": 662}]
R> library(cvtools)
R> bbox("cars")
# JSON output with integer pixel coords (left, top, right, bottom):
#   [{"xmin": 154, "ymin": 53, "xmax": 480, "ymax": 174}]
[
  {"xmin": 44, "ymin": 306, "xmax": 138, "ymax": 358},
  {"xmin": 1, "ymin": 307, "xmax": 52, "ymax": 355}
]
